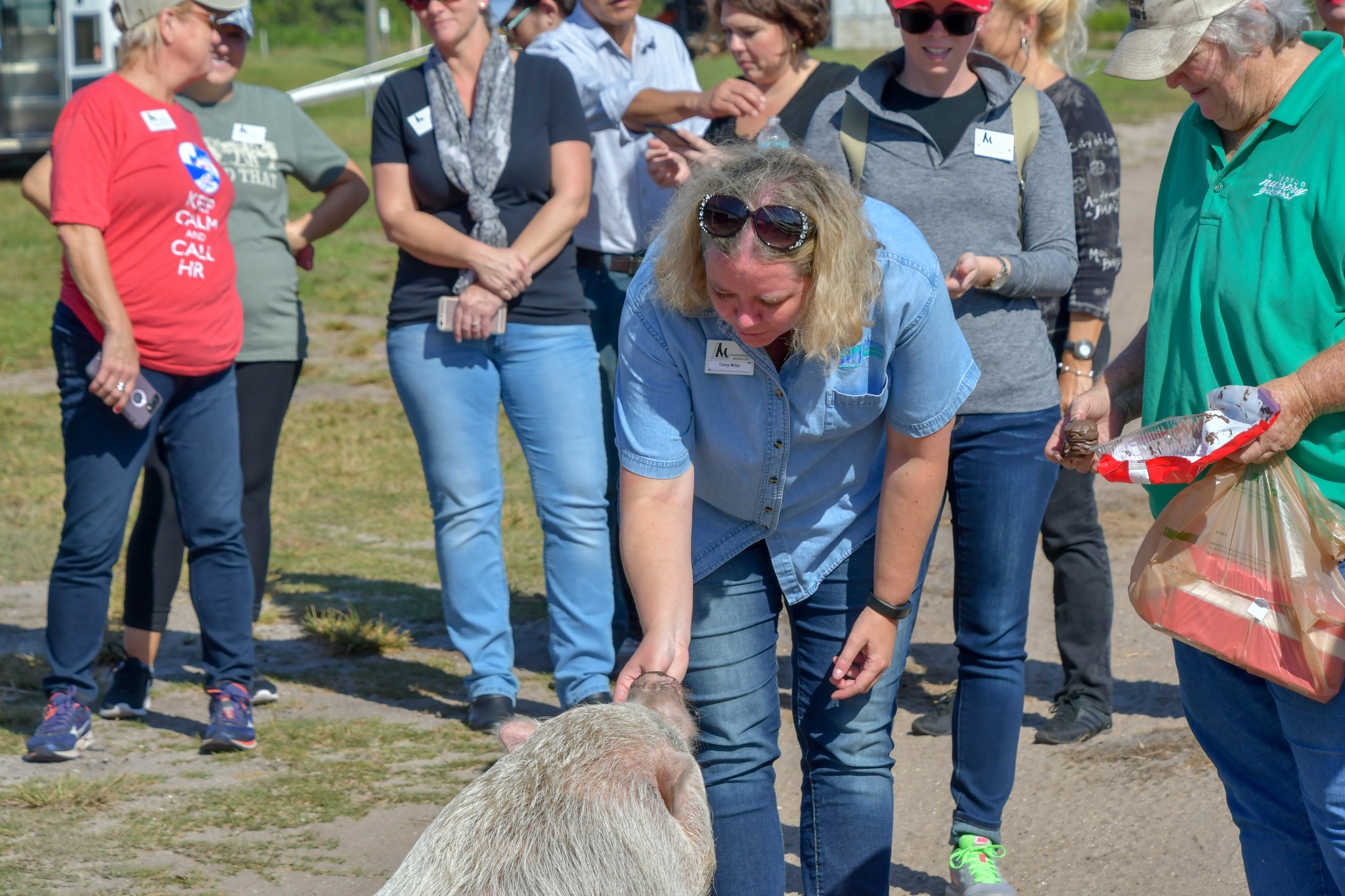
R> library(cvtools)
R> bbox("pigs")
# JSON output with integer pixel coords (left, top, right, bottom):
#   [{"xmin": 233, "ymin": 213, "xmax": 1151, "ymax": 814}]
[{"xmin": 370, "ymin": 670, "xmax": 717, "ymax": 896}]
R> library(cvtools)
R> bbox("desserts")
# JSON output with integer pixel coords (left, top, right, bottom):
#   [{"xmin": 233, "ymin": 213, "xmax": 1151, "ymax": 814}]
[{"xmin": 1062, "ymin": 419, "xmax": 1099, "ymax": 455}]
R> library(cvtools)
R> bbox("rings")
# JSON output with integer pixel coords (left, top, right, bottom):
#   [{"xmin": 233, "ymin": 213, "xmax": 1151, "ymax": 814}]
[
  {"xmin": 472, "ymin": 321, "xmax": 479, "ymax": 326},
  {"xmin": 114, "ymin": 381, "xmax": 125, "ymax": 392}
]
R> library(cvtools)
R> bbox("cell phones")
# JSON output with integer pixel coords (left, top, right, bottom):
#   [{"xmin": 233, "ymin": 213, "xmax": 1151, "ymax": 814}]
[
  {"xmin": 88, "ymin": 348, "xmax": 162, "ymax": 430},
  {"xmin": 431, "ymin": 292, "xmax": 508, "ymax": 338},
  {"xmin": 644, "ymin": 122, "xmax": 701, "ymax": 153}
]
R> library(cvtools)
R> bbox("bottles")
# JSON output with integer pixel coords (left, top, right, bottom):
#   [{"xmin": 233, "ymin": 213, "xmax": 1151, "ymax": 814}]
[{"xmin": 756, "ymin": 117, "xmax": 792, "ymax": 148}]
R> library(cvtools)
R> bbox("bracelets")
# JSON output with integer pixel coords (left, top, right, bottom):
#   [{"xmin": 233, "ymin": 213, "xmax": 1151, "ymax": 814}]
[
  {"xmin": 867, "ymin": 589, "xmax": 912, "ymax": 619},
  {"xmin": 1056, "ymin": 363, "xmax": 1095, "ymax": 378}
]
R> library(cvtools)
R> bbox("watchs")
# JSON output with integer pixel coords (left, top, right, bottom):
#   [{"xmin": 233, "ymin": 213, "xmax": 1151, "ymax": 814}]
[
  {"xmin": 1062, "ymin": 337, "xmax": 1097, "ymax": 361},
  {"xmin": 975, "ymin": 254, "xmax": 1010, "ymax": 292}
]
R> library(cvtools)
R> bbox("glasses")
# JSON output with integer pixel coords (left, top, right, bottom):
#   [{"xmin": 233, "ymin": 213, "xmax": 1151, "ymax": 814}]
[
  {"xmin": 893, "ymin": 1, "xmax": 983, "ymax": 38},
  {"xmin": 404, "ymin": 0, "xmax": 431, "ymax": 11},
  {"xmin": 697, "ymin": 192, "xmax": 817, "ymax": 253},
  {"xmin": 500, "ymin": 4, "xmax": 534, "ymax": 45}
]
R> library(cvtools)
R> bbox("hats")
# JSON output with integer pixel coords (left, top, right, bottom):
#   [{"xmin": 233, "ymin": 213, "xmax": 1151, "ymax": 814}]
[
  {"xmin": 205, "ymin": 1, "xmax": 255, "ymax": 42},
  {"xmin": 891, "ymin": 0, "xmax": 991, "ymax": 13},
  {"xmin": 1100, "ymin": 0, "xmax": 1245, "ymax": 82},
  {"xmin": 109, "ymin": 0, "xmax": 248, "ymax": 33},
  {"xmin": 482, "ymin": 0, "xmax": 516, "ymax": 30}
]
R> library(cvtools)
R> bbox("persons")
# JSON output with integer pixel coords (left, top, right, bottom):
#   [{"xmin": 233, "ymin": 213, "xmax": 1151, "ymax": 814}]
[
  {"xmin": 1313, "ymin": 0, "xmax": 1345, "ymax": 55},
  {"xmin": 526, "ymin": 0, "xmax": 707, "ymax": 681},
  {"xmin": 21, "ymin": 1, "xmax": 373, "ymax": 718},
  {"xmin": 24, "ymin": 1, "xmax": 262, "ymax": 753},
  {"xmin": 609, "ymin": 143, "xmax": 983, "ymax": 896},
  {"xmin": 809, "ymin": 0, "xmax": 1081, "ymax": 894},
  {"xmin": 1046, "ymin": 0, "xmax": 1345, "ymax": 894},
  {"xmin": 642, "ymin": 0, "xmax": 863, "ymax": 190},
  {"xmin": 369, "ymin": 0, "xmax": 613, "ymax": 733},
  {"xmin": 911, "ymin": 0, "xmax": 1126, "ymax": 747},
  {"xmin": 486, "ymin": 1, "xmax": 579, "ymax": 51}
]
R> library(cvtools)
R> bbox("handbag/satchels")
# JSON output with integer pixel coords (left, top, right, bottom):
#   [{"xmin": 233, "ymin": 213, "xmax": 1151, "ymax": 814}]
[{"xmin": 1128, "ymin": 447, "xmax": 1345, "ymax": 705}]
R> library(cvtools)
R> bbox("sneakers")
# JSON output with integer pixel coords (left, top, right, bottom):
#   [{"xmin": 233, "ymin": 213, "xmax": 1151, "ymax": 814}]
[
  {"xmin": 944, "ymin": 832, "xmax": 1017, "ymax": 896},
  {"xmin": 20, "ymin": 682, "xmax": 96, "ymax": 766},
  {"xmin": 466, "ymin": 692, "xmax": 516, "ymax": 735},
  {"xmin": 97, "ymin": 650, "xmax": 157, "ymax": 722},
  {"xmin": 197, "ymin": 681, "xmax": 261, "ymax": 752},
  {"xmin": 245, "ymin": 674, "xmax": 280, "ymax": 707},
  {"xmin": 910, "ymin": 687, "xmax": 957, "ymax": 738},
  {"xmin": 1033, "ymin": 690, "xmax": 1115, "ymax": 745}
]
